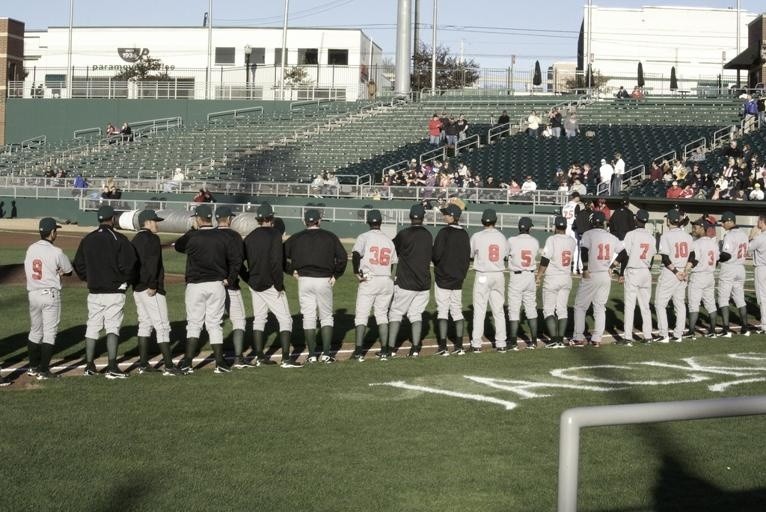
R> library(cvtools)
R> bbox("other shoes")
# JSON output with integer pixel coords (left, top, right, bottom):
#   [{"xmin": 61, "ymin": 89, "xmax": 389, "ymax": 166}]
[{"xmin": 1, "ymin": 379, "xmax": 12, "ymax": 387}]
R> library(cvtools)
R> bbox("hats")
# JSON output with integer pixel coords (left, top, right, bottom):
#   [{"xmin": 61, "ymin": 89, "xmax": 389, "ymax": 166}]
[
  {"xmin": 254, "ymin": 206, "xmax": 275, "ymax": 219},
  {"xmin": 39, "ymin": 218, "xmax": 62, "ymax": 233},
  {"xmin": 633, "ymin": 209, "xmax": 649, "ymax": 222},
  {"xmin": 671, "ymin": 181, "xmax": 678, "ymax": 186},
  {"xmin": 138, "ymin": 210, "xmax": 164, "ymax": 222},
  {"xmin": 612, "ymin": 153, "xmax": 621, "ymax": 157},
  {"xmin": 621, "ymin": 200, "xmax": 629, "ymax": 205},
  {"xmin": 554, "ymin": 216, "xmax": 567, "ymax": 228},
  {"xmin": 690, "ymin": 218, "xmax": 705, "ymax": 227},
  {"xmin": 433, "ymin": 111, "xmax": 465, "ymax": 120},
  {"xmin": 481, "ymin": 209, "xmax": 498, "ymax": 222},
  {"xmin": 190, "ymin": 206, "xmax": 212, "ymax": 219},
  {"xmin": 97, "ymin": 206, "xmax": 118, "ymax": 219},
  {"xmin": 304, "ymin": 210, "xmax": 321, "ymax": 222},
  {"xmin": 518, "ymin": 217, "xmax": 534, "ymax": 230},
  {"xmin": 410, "ymin": 207, "xmax": 425, "ymax": 219},
  {"xmin": 664, "ymin": 208, "xmax": 680, "ymax": 224},
  {"xmin": 719, "ymin": 212, "xmax": 735, "ymax": 223},
  {"xmin": 389, "ymin": 169, "xmax": 395, "ymax": 176},
  {"xmin": 367, "ymin": 210, "xmax": 382, "ymax": 222},
  {"xmin": 215, "ymin": 207, "xmax": 236, "ymax": 219},
  {"xmin": 705, "ymin": 216, "xmax": 715, "ymax": 225},
  {"xmin": 441, "ymin": 205, "xmax": 463, "ymax": 216},
  {"xmin": 571, "ymin": 192, "xmax": 579, "ymax": 198},
  {"xmin": 412, "ymin": 159, "xmax": 416, "ymax": 162},
  {"xmin": 620, "ymin": 86, "xmax": 623, "ymax": 89},
  {"xmin": 755, "ymin": 183, "xmax": 760, "ymax": 188},
  {"xmin": 593, "ymin": 211, "xmax": 605, "ymax": 223}
]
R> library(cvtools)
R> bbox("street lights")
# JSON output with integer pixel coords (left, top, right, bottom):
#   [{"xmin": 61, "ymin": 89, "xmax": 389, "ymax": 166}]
[{"xmin": 244, "ymin": 45, "xmax": 252, "ymax": 100}]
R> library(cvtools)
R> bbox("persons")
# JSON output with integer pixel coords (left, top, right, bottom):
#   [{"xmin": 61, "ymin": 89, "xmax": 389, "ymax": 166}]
[
  {"xmin": 568, "ymin": 212, "xmax": 626, "ymax": 348},
  {"xmin": 351, "ymin": 210, "xmax": 397, "ymax": 362},
  {"xmin": 630, "ymin": 86, "xmax": 644, "ymax": 109},
  {"xmin": 652, "ymin": 209, "xmax": 695, "ymax": 343},
  {"xmin": 555, "ymin": 153, "xmax": 625, "ymax": 195},
  {"xmin": 40, "ymin": 165, "xmax": 216, "ymax": 210},
  {"xmin": 717, "ymin": 211, "xmax": 751, "ymax": 337},
  {"xmin": 682, "ymin": 219, "xmax": 720, "ymax": 339},
  {"xmin": 535, "ymin": 217, "xmax": 576, "ymax": 348},
  {"xmin": 756, "ymin": 94, "xmax": 765, "ymax": 126},
  {"xmin": 282, "ymin": 209, "xmax": 347, "ymax": 364},
  {"xmin": 213, "ymin": 205, "xmax": 257, "ymax": 368},
  {"xmin": 367, "ymin": 79, "xmax": 377, "ymax": 99},
  {"xmin": 375, "ymin": 204, "xmax": 434, "ymax": 357},
  {"xmin": 496, "ymin": 175, "xmax": 536, "ymax": 203},
  {"xmin": 310, "ymin": 170, "xmax": 341, "ymax": 198},
  {"xmin": 175, "ymin": 204, "xmax": 240, "ymax": 373},
  {"xmin": 562, "ymin": 192, "xmax": 612, "ymax": 272},
  {"xmin": 239, "ymin": 205, "xmax": 305, "ymax": 369},
  {"xmin": 24, "ymin": 217, "xmax": 74, "ymax": 379},
  {"xmin": 74, "ymin": 206, "xmax": 138, "ymax": 379},
  {"xmin": 649, "ymin": 141, "xmax": 765, "ymax": 200},
  {"xmin": 745, "ymin": 95, "xmax": 757, "ymax": 117},
  {"xmin": 428, "ymin": 107, "xmax": 581, "ymax": 149},
  {"xmin": 121, "ymin": 122, "xmax": 131, "ymax": 140},
  {"xmin": 37, "ymin": 86, "xmax": 42, "ymax": 97},
  {"xmin": 615, "ymin": 87, "xmax": 629, "ymax": 109},
  {"xmin": 107, "ymin": 127, "xmax": 121, "ymax": 142},
  {"xmin": 747, "ymin": 214, "xmax": 766, "ymax": 335},
  {"xmin": 104, "ymin": 123, "xmax": 115, "ymax": 134},
  {"xmin": 31, "ymin": 83, "xmax": 35, "ymax": 98},
  {"xmin": 505, "ymin": 217, "xmax": 540, "ymax": 351},
  {"xmin": 469, "ymin": 209, "xmax": 510, "ymax": 354},
  {"xmin": 383, "ymin": 157, "xmax": 498, "ymax": 203},
  {"xmin": 130, "ymin": 210, "xmax": 187, "ymax": 376},
  {"xmin": 612, "ymin": 209, "xmax": 658, "ymax": 346},
  {"xmin": 432, "ymin": 204, "xmax": 471, "ymax": 356}
]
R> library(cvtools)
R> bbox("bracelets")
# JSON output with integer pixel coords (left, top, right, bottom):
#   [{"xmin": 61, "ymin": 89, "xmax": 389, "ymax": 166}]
[
  {"xmin": 610, "ymin": 263, "xmax": 616, "ymax": 268},
  {"xmin": 582, "ymin": 266, "xmax": 589, "ymax": 270},
  {"xmin": 671, "ymin": 267, "xmax": 678, "ymax": 274}
]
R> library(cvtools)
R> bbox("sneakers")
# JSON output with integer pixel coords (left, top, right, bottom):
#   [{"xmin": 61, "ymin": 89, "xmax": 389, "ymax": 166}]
[
  {"xmin": 611, "ymin": 327, "xmax": 763, "ymax": 347},
  {"xmin": 26, "ymin": 366, "xmax": 38, "ymax": 376},
  {"xmin": 433, "ymin": 337, "xmax": 601, "ymax": 357},
  {"xmin": 105, "ymin": 369, "xmax": 131, "ymax": 379},
  {"xmin": 83, "ymin": 367, "xmax": 100, "ymax": 377},
  {"xmin": 36, "ymin": 370, "xmax": 58, "ymax": 380},
  {"xmin": 137, "ymin": 356, "xmax": 195, "ymax": 377},
  {"xmin": 352, "ymin": 345, "xmax": 421, "ymax": 362},
  {"xmin": 213, "ymin": 353, "xmax": 336, "ymax": 373}
]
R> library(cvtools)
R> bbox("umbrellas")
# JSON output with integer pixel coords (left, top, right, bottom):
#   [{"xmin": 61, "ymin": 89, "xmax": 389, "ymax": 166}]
[
  {"xmin": 638, "ymin": 62, "xmax": 646, "ymax": 95},
  {"xmin": 671, "ymin": 67, "xmax": 679, "ymax": 96},
  {"xmin": 533, "ymin": 60, "xmax": 542, "ymax": 95},
  {"xmin": 586, "ymin": 67, "xmax": 595, "ymax": 92}
]
